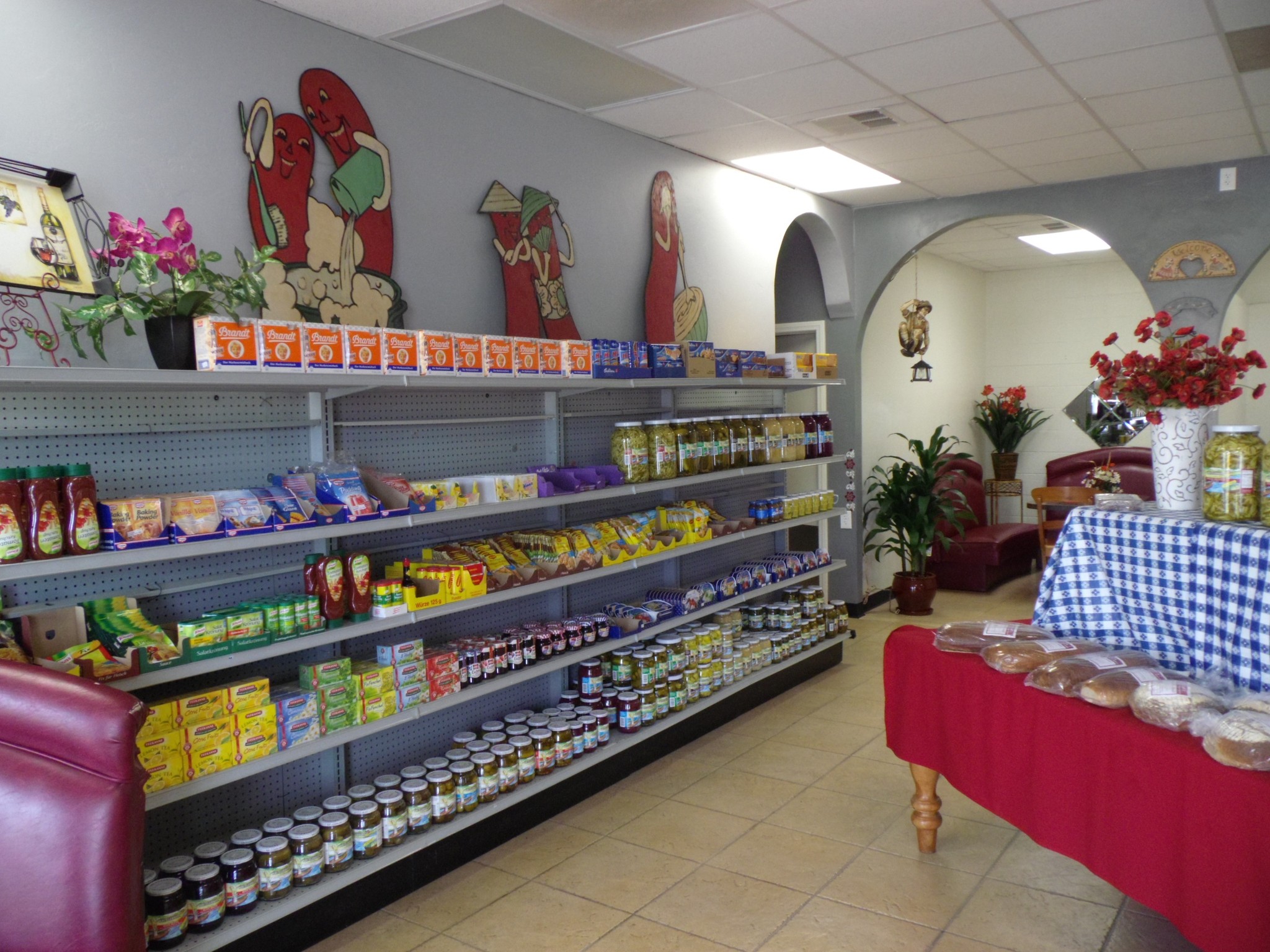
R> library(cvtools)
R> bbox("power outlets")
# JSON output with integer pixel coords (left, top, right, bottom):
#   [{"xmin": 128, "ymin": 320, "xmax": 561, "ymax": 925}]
[{"xmin": 1218, "ymin": 167, "xmax": 1237, "ymax": 192}]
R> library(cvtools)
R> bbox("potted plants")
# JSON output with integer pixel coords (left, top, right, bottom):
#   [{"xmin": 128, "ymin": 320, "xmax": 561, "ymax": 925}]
[{"xmin": 862, "ymin": 423, "xmax": 980, "ymax": 616}]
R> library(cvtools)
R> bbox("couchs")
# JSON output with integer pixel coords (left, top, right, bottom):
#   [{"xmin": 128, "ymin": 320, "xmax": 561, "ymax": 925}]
[
  {"xmin": 930, "ymin": 453, "xmax": 1040, "ymax": 594},
  {"xmin": 1044, "ymin": 446, "xmax": 1156, "ymax": 545}
]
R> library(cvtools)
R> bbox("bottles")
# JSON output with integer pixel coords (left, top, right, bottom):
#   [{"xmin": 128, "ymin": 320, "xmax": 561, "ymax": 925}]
[{"xmin": 0, "ymin": 463, "xmax": 101, "ymax": 564}]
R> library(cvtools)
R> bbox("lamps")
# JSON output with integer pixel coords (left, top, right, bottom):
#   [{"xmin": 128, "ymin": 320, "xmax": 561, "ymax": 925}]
[{"xmin": 898, "ymin": 253, "xmax": 933, "ymax": 383}]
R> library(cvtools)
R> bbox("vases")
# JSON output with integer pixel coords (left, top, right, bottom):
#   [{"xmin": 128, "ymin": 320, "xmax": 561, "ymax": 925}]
[
  {"xmin": 991, "ymin": 453, "xmax": 1019, "ymax": 481},
  {"xmin": 142, "ymin": 314, "xmax": 198, "ymax": 371},
  {"xmin": 1148, "ymin": 405, "xmax": 1221, "ymax": 512}
]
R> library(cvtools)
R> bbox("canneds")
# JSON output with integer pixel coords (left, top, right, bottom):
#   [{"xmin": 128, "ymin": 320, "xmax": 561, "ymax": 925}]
[
  {"xmin": 608, "ymin": 411, "xmax": 835, "ymax": 484},
  {"xmin": 144, "ymin": 785, "xmax": 384, "ymax": 952},
  {"xmin": 1201, "ymin": 425, "xmax": 1270, "ymax": 528},
  {"xmin": 374, "ymin": 704, "xmax": 610, "ymax": 848},
  {"xmin": 738, "ymin": 584, "xmax": 849, "ymax": 664},
  {"xmin": 748, "ymin": 489, "xmax": 834, "ymax": 525},
  {"xmin": 559, "ymin": 607, "xmax": 772, "ymax": 734},
  {"xmin": 443, "ymin": 613, "xmax": 609, "ymax": 687}
]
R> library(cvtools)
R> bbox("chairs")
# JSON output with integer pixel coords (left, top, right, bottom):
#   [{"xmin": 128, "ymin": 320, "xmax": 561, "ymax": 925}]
[{"xmin": 1030, "ymin": 485, "xmax": 1106, "ymax": 576}]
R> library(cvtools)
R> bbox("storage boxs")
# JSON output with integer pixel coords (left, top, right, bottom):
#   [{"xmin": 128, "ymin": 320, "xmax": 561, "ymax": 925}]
[
  {"xmin": 190, "ymin": 308, "xmax": 839, "ymax": 384},
  {"xmin": 129, "ymin": 606, "xmax": 462, "ymax": 797}
]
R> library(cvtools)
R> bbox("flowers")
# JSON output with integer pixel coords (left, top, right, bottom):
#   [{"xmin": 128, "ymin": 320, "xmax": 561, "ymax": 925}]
[
  {"xmin": 22, "ymin": 205, "xmax": 290, "ymax": 368},
  {"xmin": 970, "ymin": 384, "xmax": 1054, "ymax": 453},
  {"xmin": 1087, "ymin": 309, "xmax": 1269, "ymax": 427},
  {"xmin": 1074, "ymin": 450, "xmax": 1124, "ymax": 494}
]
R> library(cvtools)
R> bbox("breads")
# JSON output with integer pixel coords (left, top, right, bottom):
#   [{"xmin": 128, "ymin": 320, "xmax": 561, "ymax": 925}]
[{"xmin": 933, "ymin": 622, "xmax": 1270, "ymax": 772}]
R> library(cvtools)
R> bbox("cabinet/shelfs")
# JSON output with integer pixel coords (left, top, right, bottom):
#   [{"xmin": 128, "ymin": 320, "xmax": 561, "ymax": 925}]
[
  {"xmin": 555, "ymin": 375, "xmax": 858, "ymax": 757},
  {"xmin": 320, "ymin": 371, "xmax": 638, "ymax": 809},
  {"xmin": 0, "ymin": 363, "xmax": 420, "ymax": 952}
]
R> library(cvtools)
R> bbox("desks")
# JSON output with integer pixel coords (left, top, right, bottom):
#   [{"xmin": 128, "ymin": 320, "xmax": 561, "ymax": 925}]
[
  {"xmin": 882, "ymin": 618, "xmax": 1270, "ymax": 952},
  {"xmin": 1063, "ymin": 498, "xmax": 1270, "ymax": 697},
  {"xmin": 982, "ymin": 478, "xmax": 1024, "ymax": 526}
]
